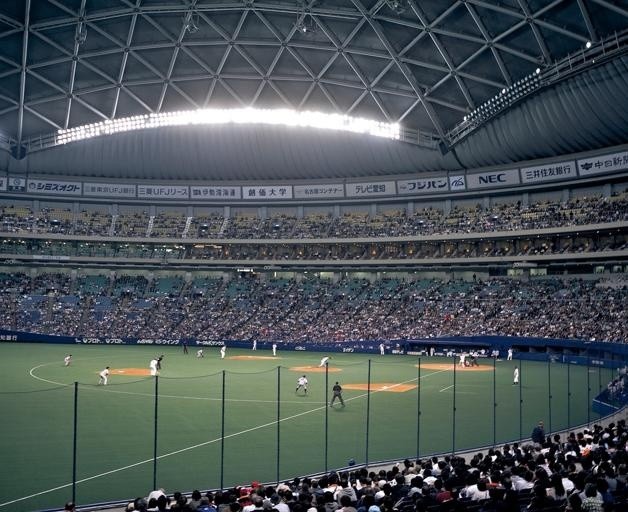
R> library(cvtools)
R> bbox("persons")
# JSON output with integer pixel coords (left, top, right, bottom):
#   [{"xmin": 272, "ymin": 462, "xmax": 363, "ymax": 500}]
[
  {"xmin": 64, "ymin": 353, "xmax": 73, "ymax": 366},
  {"xmin": 441, "ymin": 347, "xmax": 500, "ymax": 369},
  {"xmin": 271, "ymin": 342, "xmax": 277, "ymax": 356},
  {"xmin": 156, "ymin": 354, "xmax": 164, "ymax": 371},
  {"xmin": 379, "ymin": 341, "xmax": 385, "ymax": 356},
  {"xmin": 507, "ymin": 347, "xmax": 514, "ymax": 361},
  {"xmin": 511, "ymin": 365, "xmax": 519, "ymax": 386},
  {"xmin": 596, "ymin": 350, "xmax": 628, "ymax": 405},
  {"xmin": 329, "ymin": 381, "xmax": 346, "ymax": 407},
  {"xmin": 97, "ymin": 366, "xmax": 110, "ymax": 385},
  {"xmin": 196, "ymin": 349, "xmax": 204, "ymax": 359},
  {"xmin": 55, "ymin": 406, "xmax": 626, "ymax": 512},
  {"xmin": 0, "ymin": 185, "xmax": 628, "ymax": 340},
  {"xmin": 429, "ymin": 346, "xmax": 436, "ymax": 356},
  {"xmin": 318, "ymin": 354, "xmax": 331, "ymax": 368},
  {"xmin": 220, "ymin": 344, "xmax": 228, "ymax": 359},
  {"xmin": 150, "ymin": 356, "xmax": 161, "ymax": 377},
  {"xmin": 252, "ymin": 337, "xmax": 257, "ymax": 350},
  {"xmin": 182, "ymin": 340, "xmax": 189, "ymax": 355},
  {"xmin": 295, "ymin": 374, "xmax": 308, "ymax": 394}
]
